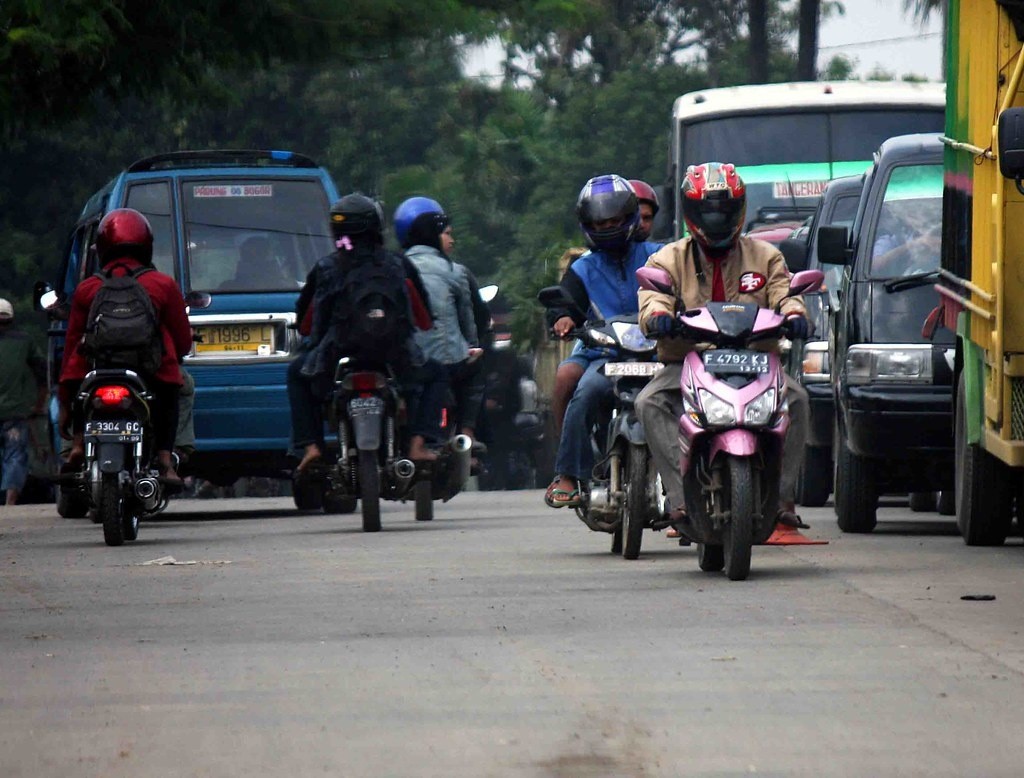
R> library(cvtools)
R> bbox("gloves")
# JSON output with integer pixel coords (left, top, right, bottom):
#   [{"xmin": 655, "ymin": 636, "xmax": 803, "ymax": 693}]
[
  {"xmin": 646, "ymin": 312, "xmax": 674, "ymax": 338},
  {"xmin": 785, "ymin": 312, "xmax": 808, "ymax": 341},
  {"xmin": 58, "ymin": 402, "xmax": 72, "ymax": 442}
]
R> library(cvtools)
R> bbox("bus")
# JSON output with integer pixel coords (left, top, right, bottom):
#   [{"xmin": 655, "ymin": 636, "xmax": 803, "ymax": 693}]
[{"xmin": 647, "ymin": 75, "xmax": 955, "ymax": 236}]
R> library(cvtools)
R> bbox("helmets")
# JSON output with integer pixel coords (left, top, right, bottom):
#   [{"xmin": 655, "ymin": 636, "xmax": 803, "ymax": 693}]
[
  {"xmin": 679, "ymin": 162, "xmax": 746, "ymax": 253},
  {"xmin": 627, "ymin": 179, "xmax": 659, "ymax": 218},
  {"xmin": 96, "ymin": 208, "xmax": 155, "ymax": 265},
  {"xmin": 328, "ymin": 192, "xmax": 387, "ymax": 245},
  {"xmin": 392, "ymin": 196, "xmax": 446, "ymax": 245},
  {"xmin": 573, "ymin": 174, "xmax": 641, "ymax": 254},
  {"xmin": 0, "ymin": 297, "xmax": 13, "ymax": 318}
]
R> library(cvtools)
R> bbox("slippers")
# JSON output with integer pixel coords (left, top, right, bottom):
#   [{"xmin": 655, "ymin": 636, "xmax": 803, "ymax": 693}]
[
  {"xmin": 655, "ymin": 509, "xmax": 689, "ymax": 527},
  {"xmin": 552, "ymin": 488, "xmax": 579, "ymax": 507},
  {"xmin": 777, "ymin": 505, "xmax": 811, "ymax": 529}
]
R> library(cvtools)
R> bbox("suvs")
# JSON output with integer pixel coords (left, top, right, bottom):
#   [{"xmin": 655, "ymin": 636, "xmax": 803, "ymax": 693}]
[{"xmin": 778, "ymin": 130, "xmax": 961, "ymax": 536}]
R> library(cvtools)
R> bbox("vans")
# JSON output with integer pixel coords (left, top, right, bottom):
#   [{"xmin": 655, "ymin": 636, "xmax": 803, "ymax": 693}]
[{"xmin": 43, "ymin": 147, "xmax": 359, "ymax": 515}]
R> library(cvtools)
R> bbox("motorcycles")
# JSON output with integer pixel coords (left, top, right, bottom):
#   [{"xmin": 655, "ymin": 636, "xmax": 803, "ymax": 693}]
[
  {"xmin": 633, "ymin": 266, "xmax": 828, "ymax": 581},
  {"xmin": 40, "ymin": 285, "xmax": 214, "ymax": 547},
  {"xmin": 394, "ymin": 282, "xmax": 503, "ymax": 521},
  {"xmin": 283, "ymin": 310, "xmax": 445, "ymax": 531},
  {"xmin": 537, "ymin": 284, "xmax": 667, "ymax": 560}
]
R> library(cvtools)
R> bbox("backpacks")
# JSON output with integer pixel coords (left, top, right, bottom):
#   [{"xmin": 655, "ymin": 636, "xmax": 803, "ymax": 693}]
[
  {"xmin": 81, "ymin": 266, "xmax": 167, "ymax": 369},
  {"xmin": 330, "ymin": 249, "xmax": 411, "ymax": 372}
]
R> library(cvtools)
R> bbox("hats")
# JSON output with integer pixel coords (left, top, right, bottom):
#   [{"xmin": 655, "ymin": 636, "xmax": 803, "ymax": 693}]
[{"xmin": 407, "ymin": 211, "xmax": 450, "ymax": 249}]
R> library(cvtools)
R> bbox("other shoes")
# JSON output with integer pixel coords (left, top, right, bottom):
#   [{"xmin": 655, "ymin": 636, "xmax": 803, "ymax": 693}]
[
  {"xmin": 65, "ymin": 430, "xmax": 85, "ymax": 466},
  {"xmin": 545, "ymin": 475, "xmax": 563, "ymax": 508},
  {"xmin": 471, "ymin": 438, "xmax": 486, "ymax": 452},
  {"xmin": 156, "ymin": 462, "xmax": 181, "ymax": 484}
]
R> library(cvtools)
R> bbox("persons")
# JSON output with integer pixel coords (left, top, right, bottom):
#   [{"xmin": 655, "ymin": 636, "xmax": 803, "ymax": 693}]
[
  {"xmin": 285, "ymin": 195, "xmax": 446, "ymax": 471},
  {"xmin": 219, "ymin": 236, "xmax": 298, "ymax": 290},
  {"xmin": 545, "ymin": 174, "xmax": 668, "ymax": 509},
  {"xmin": 514, "ymin": 357, "xmax": 541, "ymax": 427},
  {"xmin": 57, "ymin": 207, "xmax": 193, "ymax": 482},
  {"xmin": 639, "ymin": 160, "xmax": 811, "ymax": 529},
  {"xmin": 0, "ymin": 297, "xmax": 47, "ymax": 505},
  {"xmin": 393, "ymin": 197, "xmax": 493, "ymax": 450},
  {"xmin": 871, "ymin": 204, "xmax": 941, "ymax": 272}
]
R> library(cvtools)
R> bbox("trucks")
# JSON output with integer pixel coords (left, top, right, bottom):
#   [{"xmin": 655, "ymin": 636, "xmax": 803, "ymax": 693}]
[{"xmin": 929, "ymin": 0, "xmax": 1024, "ymax": 548}]
